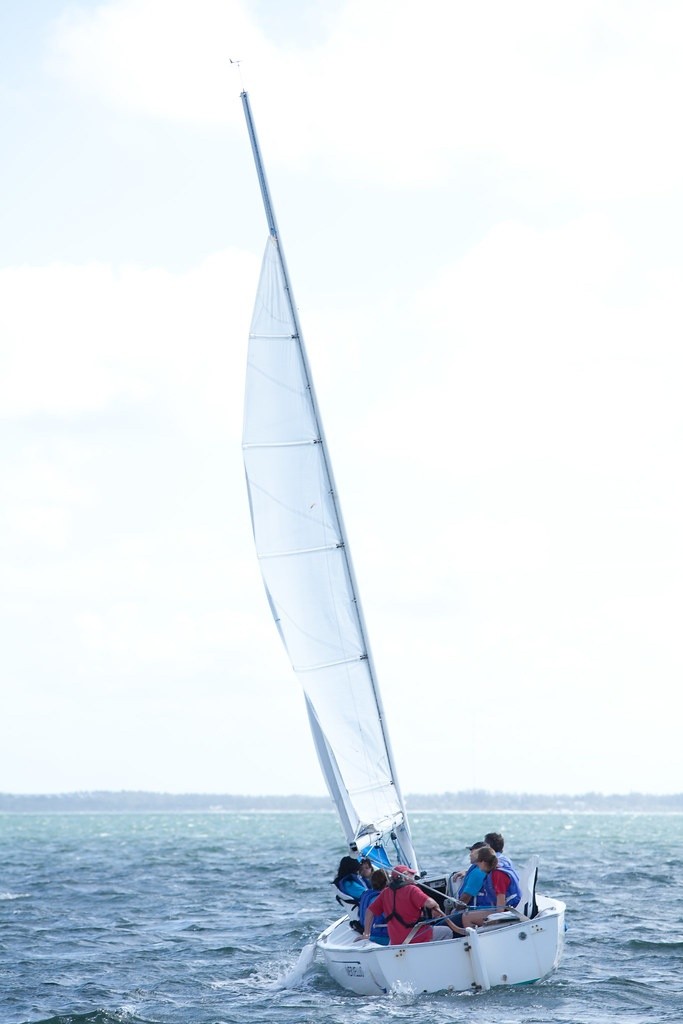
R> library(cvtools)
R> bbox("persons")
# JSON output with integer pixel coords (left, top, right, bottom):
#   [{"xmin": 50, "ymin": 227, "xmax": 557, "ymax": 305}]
[
  {"xmin": 452, "ymin": 832, "xmax": 522, "ymax": 929},
  {"xmin": 334, "ymin": 856, "xmax": 465, "ymax": 946}
]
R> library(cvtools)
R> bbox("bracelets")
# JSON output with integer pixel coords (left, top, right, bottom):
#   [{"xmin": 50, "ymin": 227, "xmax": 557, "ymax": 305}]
[{"xmin": 363, "ymin": 932, "xmax": 370, "ymax": 936}]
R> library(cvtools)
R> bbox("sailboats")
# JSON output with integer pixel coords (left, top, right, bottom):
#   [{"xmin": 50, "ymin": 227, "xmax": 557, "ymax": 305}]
[{"xmin": 229, "ymin": 88, "xmax": 570, "ymax": 1008}]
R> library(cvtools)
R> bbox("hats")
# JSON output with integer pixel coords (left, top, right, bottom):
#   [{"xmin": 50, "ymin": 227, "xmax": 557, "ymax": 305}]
[
  {"xmin": 466, "ymin": 842, "xmax": 488, "ymax": 850},
  {"xmin": 392, "ymin": 866, "xmax": 417, "ymax": 878},
  {"xmin": 338, "ymin": 856, "xmax": 360, "ymax": 875}
]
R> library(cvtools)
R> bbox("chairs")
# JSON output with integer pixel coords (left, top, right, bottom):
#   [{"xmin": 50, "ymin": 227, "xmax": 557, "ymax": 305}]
[
  {"xmin": 484, "ymin": 867, "xmax": 539, "ymax": 924},
  {"xmin": 330, "ymin": 884, "xmax": 361, "ymax": 930}
]
